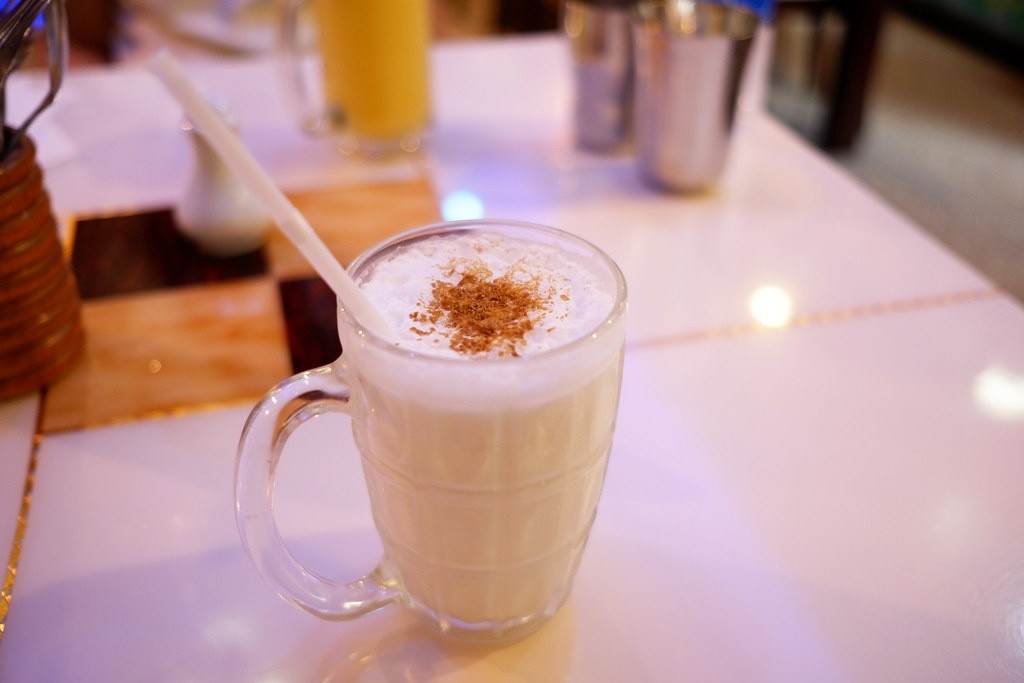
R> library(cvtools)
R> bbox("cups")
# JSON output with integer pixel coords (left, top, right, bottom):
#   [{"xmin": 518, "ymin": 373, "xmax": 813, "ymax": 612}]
[
  {"xmin": 629, "ymin": 0, "xmax": 772, "ymax": 199},
  {"xmin": 233, "ymin": 219, "xmax": 628, "ymax": 647},
  {"xmin": 563, "ymin": 1, "xmax": 637, "ymax": 159},
  {"xmin": 283, "ymin": 1, "xmax": 429, "ymax": 159},
  {"xmin": 1, "ymin": 128, "xmax": 85, "ymax": 397}
]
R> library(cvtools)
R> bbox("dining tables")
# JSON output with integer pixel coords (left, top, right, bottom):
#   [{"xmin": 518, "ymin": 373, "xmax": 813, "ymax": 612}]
[{"xmin": 2, "ymin": 35, "xmax": 1024, "ymax": 683}]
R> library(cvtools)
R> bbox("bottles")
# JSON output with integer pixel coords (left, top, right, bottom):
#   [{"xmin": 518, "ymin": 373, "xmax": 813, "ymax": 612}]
[{"xmin": 173, "ymin": 116, "xmax": 273, "ymax": 254}]
